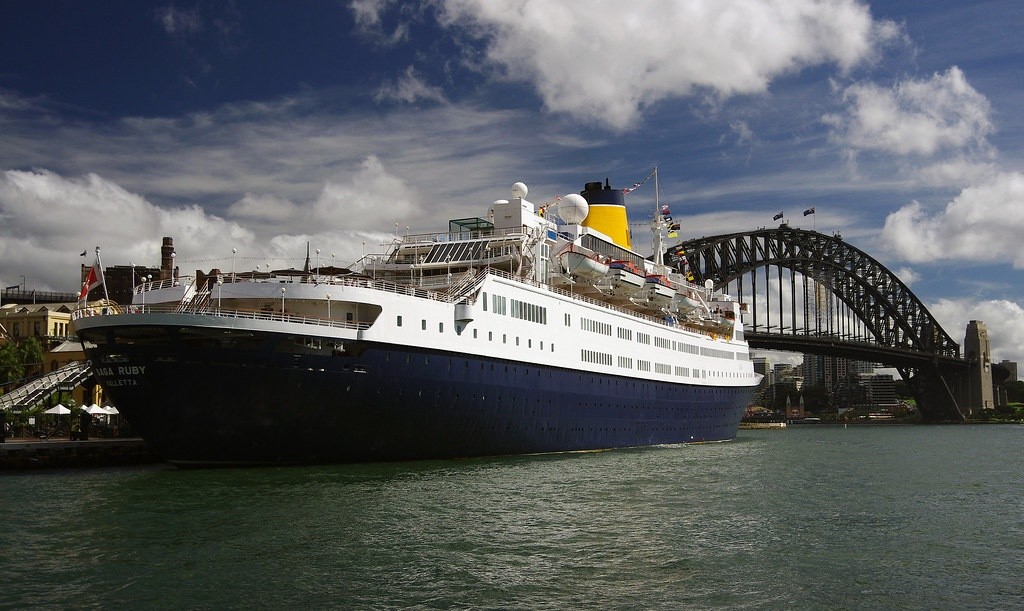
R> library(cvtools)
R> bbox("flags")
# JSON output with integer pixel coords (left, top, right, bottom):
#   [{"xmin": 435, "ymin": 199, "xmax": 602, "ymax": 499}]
[
  {"xmin": 661, "ymin": 203, "xmax": 694, "ymax": 281},
  {"xmin": 80, "ymin": 259, "xmax": 102, "ymax": 300},
  {"xmin": 773, "ymin": 211, "xmax": 783, "ymax": 221},
  {"xmin": 804, "ymin": 207, "xmax": 815, "ymax": 216},
  {"xmin": 621, "ymin": 183, "xmax": 640, "ymax": 195}
]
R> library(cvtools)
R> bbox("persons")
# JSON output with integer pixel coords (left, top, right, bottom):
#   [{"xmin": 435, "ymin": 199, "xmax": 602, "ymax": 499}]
[{"xmin": 70, "ymin": 422, "xmax": 80, "ymax": 442}]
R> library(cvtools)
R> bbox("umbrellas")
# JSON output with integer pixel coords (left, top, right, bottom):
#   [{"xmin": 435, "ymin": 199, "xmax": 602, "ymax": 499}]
[
  {"xmin": 41, "ymin": 404, "xmax": 71, "ymax": 429},
  {"xmin": 79, "ymin": 403, "xmax": 120, "ymax": 425}
]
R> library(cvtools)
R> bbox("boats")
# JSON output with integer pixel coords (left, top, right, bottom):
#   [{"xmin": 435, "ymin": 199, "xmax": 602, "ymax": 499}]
[{"xmin": 71, "ymin": 177, "xmax": 765, "ymax": 467}]
